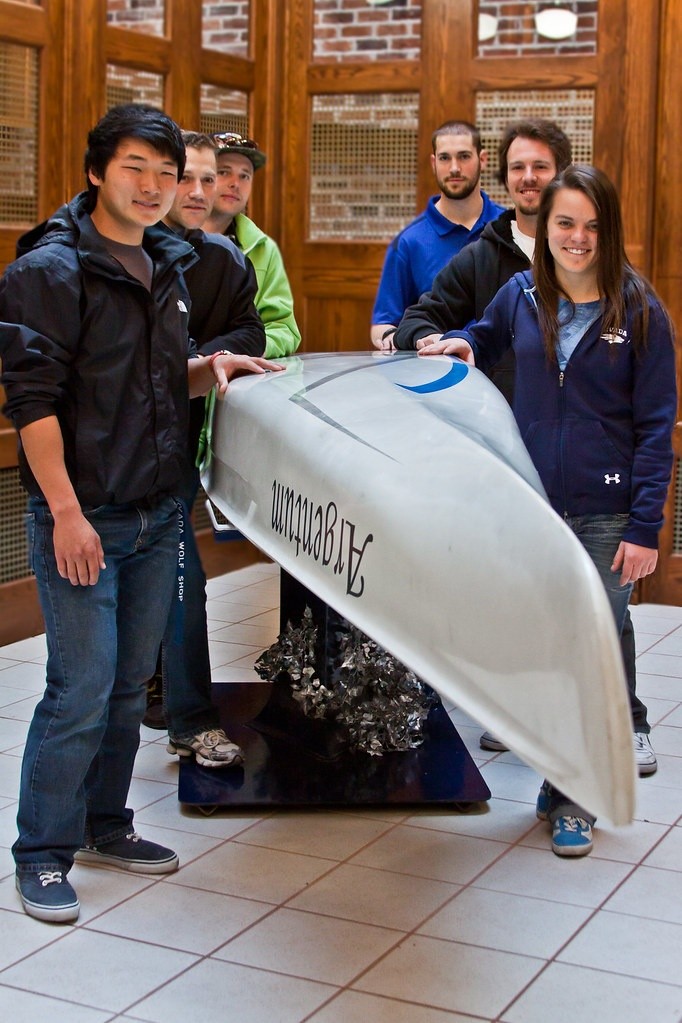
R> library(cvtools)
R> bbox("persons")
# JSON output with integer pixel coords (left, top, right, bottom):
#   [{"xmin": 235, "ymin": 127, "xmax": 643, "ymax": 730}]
[
  {"xmin": 14, "ymin": 131, "xmax": 266, "ymax": 768},
  {"xmin": 392, "ymin": 119, "xmax": 657, "ymax": 772},
  {"xmin": 417, "ymin": 164, "xmax": 678, "ymax": 855},
  {"xmin": 0, "ymin": 102, "xmax": 286, "ymax": 922},
  {"xmin": 370, "ymin": 121, "xmax": 509, "ymax": 351},
  {"xmin": 143, "ymin": 132, "xmax": 302, "ymax": 730}
]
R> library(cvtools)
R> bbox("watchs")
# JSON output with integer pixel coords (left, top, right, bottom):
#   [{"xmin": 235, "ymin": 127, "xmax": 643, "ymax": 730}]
[{"xmin": 382, "ymin": 327, "xmax": 397, "ymax": 341}]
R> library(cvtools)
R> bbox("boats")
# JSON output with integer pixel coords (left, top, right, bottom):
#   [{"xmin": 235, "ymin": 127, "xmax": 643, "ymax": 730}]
[{"xmin": 181, "ymin": 346, "xmax": 640, "ymax": 830}]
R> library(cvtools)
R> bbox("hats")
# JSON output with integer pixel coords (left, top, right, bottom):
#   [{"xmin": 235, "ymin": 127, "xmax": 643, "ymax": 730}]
[{"xmin": 206, "ymin": 130, "xmax": 267, "ymax": 169}]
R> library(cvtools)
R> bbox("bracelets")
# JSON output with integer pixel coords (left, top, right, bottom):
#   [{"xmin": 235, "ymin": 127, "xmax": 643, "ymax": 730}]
[{"xmin": 209, "ymin": 350, "xmax": 232, "ymax": 372}]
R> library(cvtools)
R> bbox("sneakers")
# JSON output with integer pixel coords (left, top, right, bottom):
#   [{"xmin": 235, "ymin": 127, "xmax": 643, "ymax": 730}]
[
  {"xmin": 551, "ymin": 815, "xmax": 593, "ymax": 855},
  {"xmin": 633, "ymin": 732, "xmax": 658, "ymax": 773},
  {"xmin": 71, "ymin": 831, "xmax": 181, "ymax": 874},
  {"xmin": 13, "ymin": 863, "xmax": 81, "ymax": 921},
  {"xmin": 164, "ymin": 727, "xmax": 248, "ymax": 767},
  {"xmin": 479, "ymin": 731, "xmax": 511, "ymax": 751},
  {"xmin": 141, "ymin": 651, "xmax": 168, "ymax": 731},
  {"xmin": 535, "ymin": 778, "xmax": 567, "ymax": 820}
]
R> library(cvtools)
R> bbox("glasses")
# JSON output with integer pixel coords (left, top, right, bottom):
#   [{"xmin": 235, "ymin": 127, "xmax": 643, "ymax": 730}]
[{"xmin": 214, "ymin": 132, "xmax": 258, "ymax": 150}]
